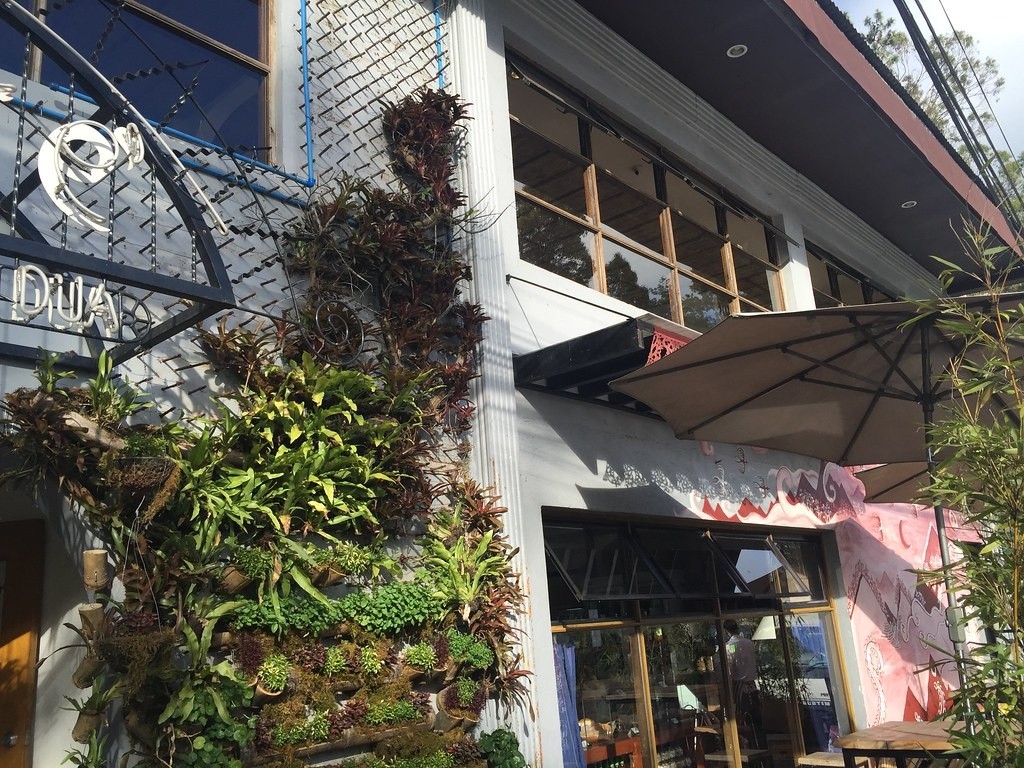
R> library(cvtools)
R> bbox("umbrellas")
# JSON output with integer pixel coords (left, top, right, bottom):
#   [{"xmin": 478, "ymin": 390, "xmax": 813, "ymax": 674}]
[
  {"xmin": 607, "ymin": 293, "xmax": 1024, "ymax": 739},
  {"xmin": 845, "ymin": 447, "xmax": 996, "ymax": 513}
]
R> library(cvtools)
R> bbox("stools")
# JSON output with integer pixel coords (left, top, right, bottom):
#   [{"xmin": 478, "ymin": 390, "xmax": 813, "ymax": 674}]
[{"xmin": 797, "ymin": 752, "xmax": 872, "ymax": 768}]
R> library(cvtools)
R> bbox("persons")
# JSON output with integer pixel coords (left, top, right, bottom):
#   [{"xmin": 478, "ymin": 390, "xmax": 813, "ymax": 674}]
[{"xmin": 722, "ymin": 619, "xmax": 773, "ymax": 765}]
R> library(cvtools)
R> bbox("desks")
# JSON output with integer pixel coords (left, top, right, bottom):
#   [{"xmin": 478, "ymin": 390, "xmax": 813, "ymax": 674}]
[{"xmin": 832, "ymin": 720, "xmax": 1024, "ymax": 768}]
